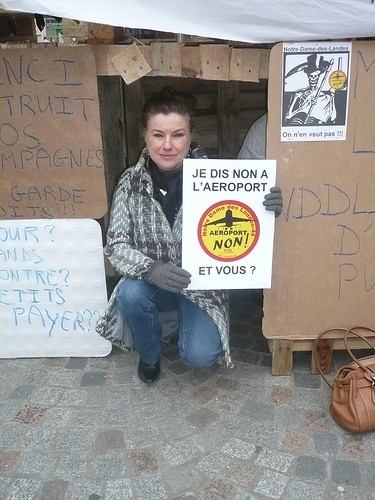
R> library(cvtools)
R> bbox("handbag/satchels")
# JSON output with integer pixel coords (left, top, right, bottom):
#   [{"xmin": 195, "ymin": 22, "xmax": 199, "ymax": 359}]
[{"xmin": 313, "ymin": 325, "xmax": 375, "ymax": 434}]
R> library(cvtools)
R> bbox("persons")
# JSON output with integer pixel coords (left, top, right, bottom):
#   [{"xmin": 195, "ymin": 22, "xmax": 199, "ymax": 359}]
[
  {"xmin": 94, "ymin": 88, "xmax": 284, "ymax": 384},
  {"xmin": 239, "ymin": 112, "xmax": 267, "ymax": 161}
]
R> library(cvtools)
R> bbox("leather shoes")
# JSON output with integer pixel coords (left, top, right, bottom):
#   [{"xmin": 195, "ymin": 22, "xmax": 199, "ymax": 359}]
[{"xmin": 136, "ymin": 356, "xmax": 160, "ymax": 384}]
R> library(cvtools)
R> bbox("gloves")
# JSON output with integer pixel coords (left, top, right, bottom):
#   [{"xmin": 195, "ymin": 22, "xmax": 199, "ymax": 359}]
[
  {"xmin": 263, "ymin": 186, "xmax": 282, "ymax": 217},
  {"xmin": 143, "ymin": 259, "xmax": 192, "ymax": 294}
]
